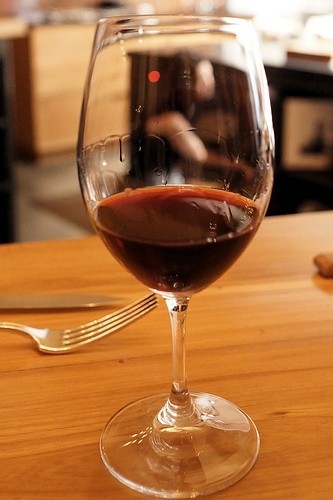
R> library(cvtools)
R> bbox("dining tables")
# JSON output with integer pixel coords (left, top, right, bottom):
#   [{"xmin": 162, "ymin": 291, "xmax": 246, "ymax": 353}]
[{"xmin": 0, "ymin": 208, "xmax": 333, "ymax": 500}]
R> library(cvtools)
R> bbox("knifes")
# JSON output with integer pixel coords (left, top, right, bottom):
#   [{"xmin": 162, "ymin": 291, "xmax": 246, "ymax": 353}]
[{"xmin": 0, "ymin": 292, "xmax": 131, "ymax": 310}]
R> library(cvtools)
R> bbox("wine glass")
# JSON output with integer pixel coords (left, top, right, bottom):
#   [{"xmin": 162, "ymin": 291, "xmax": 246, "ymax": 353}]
[{"xmin": 76, "ymin": 13, "xmax": 274, "ymax": 499}]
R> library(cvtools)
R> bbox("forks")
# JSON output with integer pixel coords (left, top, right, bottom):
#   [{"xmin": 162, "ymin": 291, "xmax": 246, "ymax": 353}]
[{"xmin": 0, "ymin": 290, "xmax": 158, "ymax": 355}]
